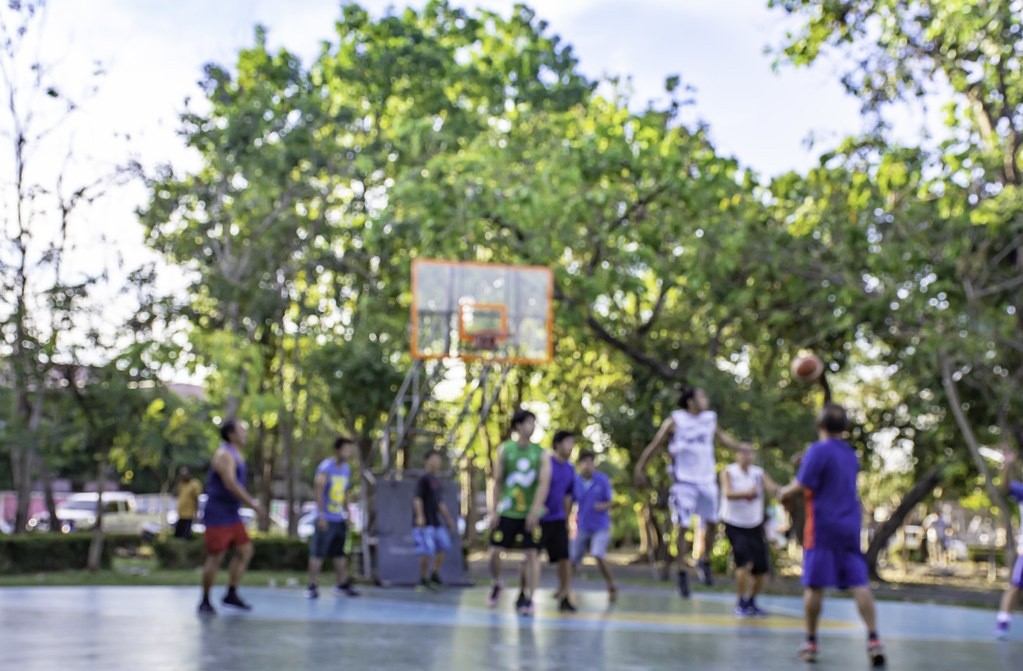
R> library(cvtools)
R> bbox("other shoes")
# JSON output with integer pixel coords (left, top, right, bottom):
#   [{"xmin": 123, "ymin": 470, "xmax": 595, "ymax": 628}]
[
  {"xmin": 516, "ymin": 595, "xmax": 534, "ymax": 616},
  {"xmin": 418, "ymin": 580, "xmax": 435, "ymax": 592},
  {"xmin": 490, "ymin": 583, "xmax": 500, "ymax": 600},
  {"xmin": 223, "ymin": 594, "xmax": 249, "ymax": 610},
  {"xmin": 867, "ymin": 638, "xmax": 885, "ymax": 669},
  {"xmin": 996, "ymin": 617, "xmax": 1010, "ymax": 634},
  {"xmin": 310, "ymin": 587, "xmax": 320, "ymax": 599},
  {"xmin": 798, "ymin": 642, "xmax": 818, "ymax": 661},
  {"xmin": 559, "ymin": 597, "xmax": 574, "ymax": 612},
  {"xmin": 339, "ymin": 582, "xmax": 361, "ymax": 598},
  {"xmin": 679, "ymin": 573, "xmax": 690, "ymax": 599},
  {"xmin": 199, "ymin": 601, "xmax": 215, "ymax": 613},
  {"xmin": 692, "ymin": 557, "xmax": 712, "ymax": 587},
  {"xmin": 428, "ymin": 573, "xmax": 444, "ymax": 587},
  {"xmin": 735, "ymin": 603, "xmax": 766, "ymax": 616},
  {"xmin": 607, "ymin": 586, "xmax": 616, "ymax": 603}
]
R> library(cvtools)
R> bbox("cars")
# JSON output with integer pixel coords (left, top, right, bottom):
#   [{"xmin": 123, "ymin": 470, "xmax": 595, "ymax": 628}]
[{"xmin": 134, "ymin": 493, "xmax": 319, "ymax": 539}]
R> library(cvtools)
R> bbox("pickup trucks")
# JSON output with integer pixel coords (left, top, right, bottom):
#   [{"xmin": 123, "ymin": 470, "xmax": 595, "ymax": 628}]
[{"xmin": 30, "ymin": 490, "xmax": 166, "ymax": 535}]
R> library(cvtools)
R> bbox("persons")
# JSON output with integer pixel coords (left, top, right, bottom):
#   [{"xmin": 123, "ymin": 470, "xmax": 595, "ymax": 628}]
[
  {"xmin": 198, "ymin": 417, "xmax": 275, "ymax": 613},
  {"xmin": 779, "ymin": 404, "xmax": 886, "ymax": 667},
  {"xmin": 555, "ymin": 450, "xmax": 618, "ymax": 601},
  {"xmin": 719, "ymin": 438, "xmax": 778, "ymax": 616},
  {"xmin": 304, "ymin": 437, "xmax": 360, "ymax": 599},
  {"xmin": 519, "ymin": 431, "xmax": 578, "ymax": 614},
  {"xmin": 631, "ymin": 386, "xmax": 753, "ymax": 596},
  {"xmin": 994, "ymin": 449, "xmax": 1023, "ymax": 632},
  {"xmin": 486, "ymin": 410, "xmax": 554, "ymax": 611},
  {"xmin": 410, "ymin": 451, "xmax": 457, "ymax": 594},
  {"xmin": 171, "ymin": 466, "xmax": 203, "ymax": 540}
]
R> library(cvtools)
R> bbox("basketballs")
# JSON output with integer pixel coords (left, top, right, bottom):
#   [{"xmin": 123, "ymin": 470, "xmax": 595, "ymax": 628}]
[{"xmin": 790, "ymin": 353, "xmax": 825, "ymax": 385}]
